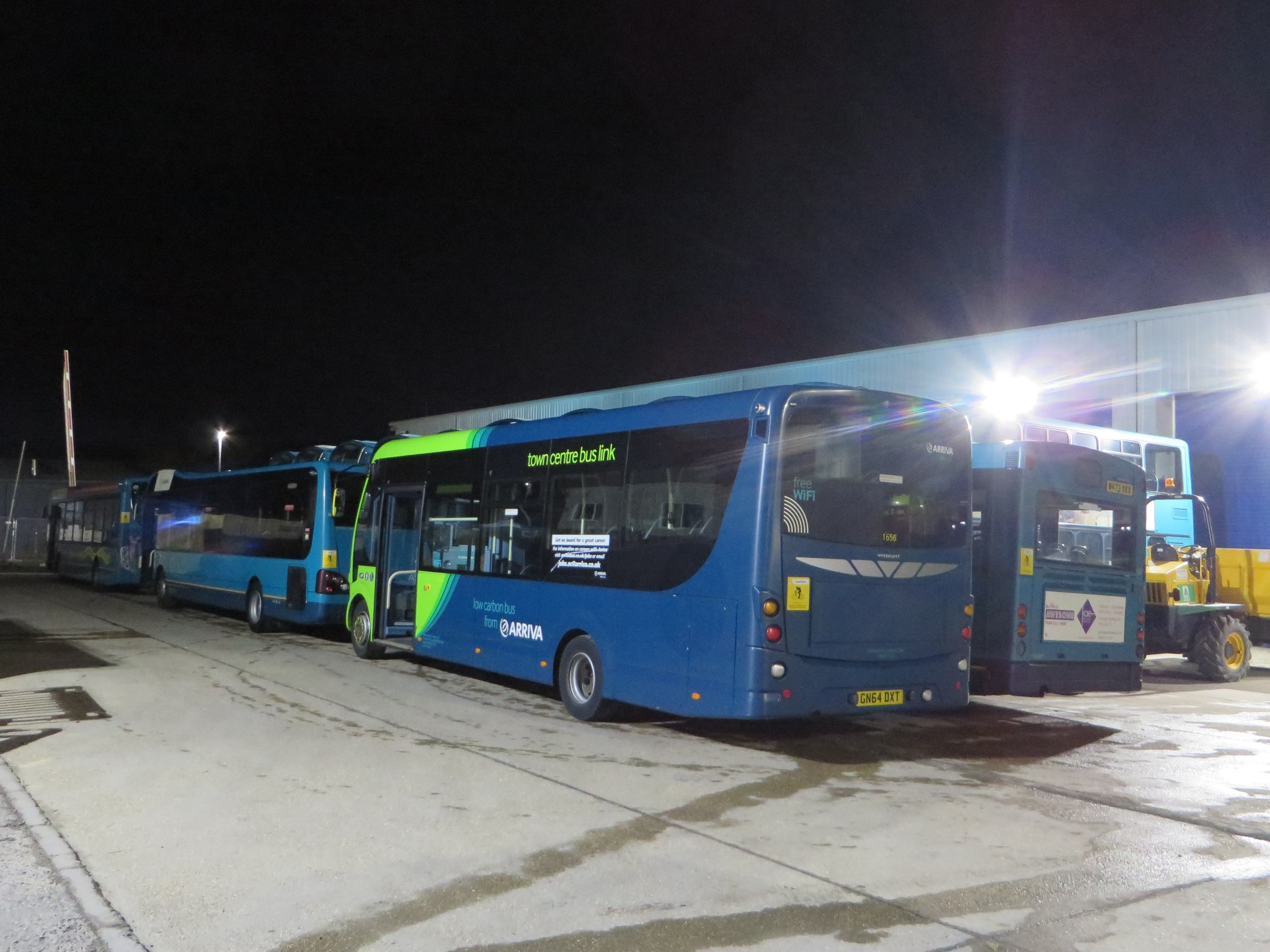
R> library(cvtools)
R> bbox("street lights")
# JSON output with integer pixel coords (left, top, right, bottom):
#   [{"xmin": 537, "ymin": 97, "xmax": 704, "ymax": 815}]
[{"xmin": 214, "ymin": 429, "xmax": 228, "ymax": 472}]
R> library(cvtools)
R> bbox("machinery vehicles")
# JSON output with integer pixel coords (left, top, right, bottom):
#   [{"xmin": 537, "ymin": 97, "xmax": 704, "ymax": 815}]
[{"xmin": 1113, "ymin": 492, "xmax": 1253, "ymax": 682}]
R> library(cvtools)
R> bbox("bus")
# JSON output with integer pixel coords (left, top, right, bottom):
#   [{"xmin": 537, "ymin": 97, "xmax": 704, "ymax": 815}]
[
  {"xmin": 968, "ymin": 441, "xmax": 1146, "ymax": 697},
  {"xmin": 248, "ymin": 395, "xmax": 694, "ymax": 467},
  {"xmin": 332, "ymin": 381, "xmax": 974, "ymax": 722},
  {"xmin": 945, "ymin": 404, "xmax": 1195, "ymax": 565},
  {"xmin": 133, "ymin": 460, "xmax": 380, "ymax": 632},
  {"xmin": 42, "ymin": 477, "xmax": 152, "ymax": 585}
]
